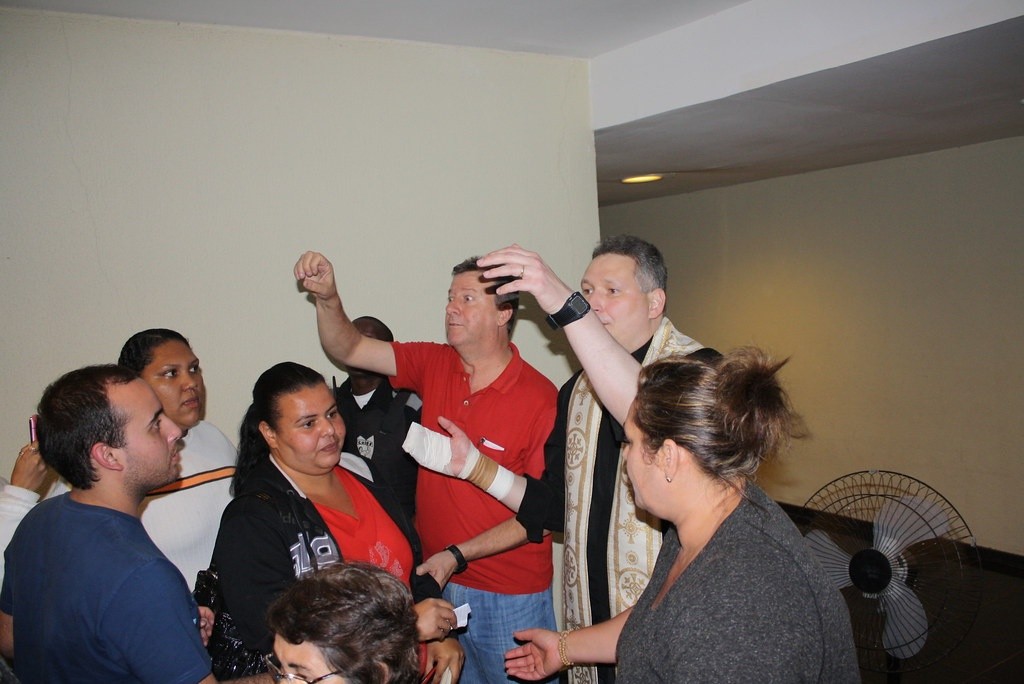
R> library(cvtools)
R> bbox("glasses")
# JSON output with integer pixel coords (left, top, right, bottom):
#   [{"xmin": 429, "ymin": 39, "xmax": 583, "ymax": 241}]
[{"xmin": 266, "ymin": 652, "xmax": 343, "ymax": 684}]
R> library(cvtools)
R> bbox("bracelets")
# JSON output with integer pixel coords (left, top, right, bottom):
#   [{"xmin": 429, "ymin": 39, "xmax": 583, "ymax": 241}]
[{"xmin": 559, "ymin": 629, "xmax": 575, "ymax": 666}]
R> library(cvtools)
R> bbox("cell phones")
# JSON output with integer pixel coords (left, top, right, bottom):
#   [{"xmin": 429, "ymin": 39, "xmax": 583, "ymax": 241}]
[{"xmin": 29, "ymin": 414, "xmax": 38, "ymax": 444}]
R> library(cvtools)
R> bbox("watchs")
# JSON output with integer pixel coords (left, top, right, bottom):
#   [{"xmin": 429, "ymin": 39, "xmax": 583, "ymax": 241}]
[
  {"xmin": 546, "ymin": 290, "xmax": 591, "ymax": 330},
  {"xmin": 443, "ymin": 544, "xmax": 468, "ymax": 573}
]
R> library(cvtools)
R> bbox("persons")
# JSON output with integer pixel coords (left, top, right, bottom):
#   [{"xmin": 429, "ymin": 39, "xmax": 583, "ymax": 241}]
[
  {"xmin": 203, "ymin": 358, "xmax": 466, "ymax": 684},
  {"xmin": 329, "ymin": 315, "xmax": 423, "ymax": 537},
  {"xmin": 502, "ymin": 344, "xmax": 862, "ymax": 684},
  {"xmin": 294, "ymin": 250, "xmax": 567, "ymax": 684},
  {"xmin": 401, "ymin": 234, "xmax": 722, "ymax": 684},
  {"xmin": 0, "ymin": 438, "xmax": 48, "ymax": 598},
  {"xmin": 54, "ymin": 327, "xmax": 239, "ymax": 591},
  {"xmin": 265, "ymin": 558, "xmax": 421, "ymax": 684},
  {"xmin": 0, "ymin": 363, "xmax": 274, "ymax": 684}
]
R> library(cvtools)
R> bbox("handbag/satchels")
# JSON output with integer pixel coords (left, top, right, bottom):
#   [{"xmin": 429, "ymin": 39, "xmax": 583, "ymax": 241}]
[{"xmin": 193, "ymin": 491, "xmax": 317, "ymax": 682}]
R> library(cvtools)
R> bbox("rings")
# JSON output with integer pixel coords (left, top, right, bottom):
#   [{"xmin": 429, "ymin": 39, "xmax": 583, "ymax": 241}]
[
  {"xmin": 29, "ymin": 446, "xmax": 37, "ymax": 454},
  {"xmin": 19, "ymin": 452, "xmax": 24, "ymax": 456},
  {"xmin": 520, "ymin": 265, "xmax": 525, "ymax": 277}
]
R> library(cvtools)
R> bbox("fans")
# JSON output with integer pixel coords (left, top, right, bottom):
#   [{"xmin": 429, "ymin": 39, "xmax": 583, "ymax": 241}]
[{"xmin": 796, "ymin": 469, "xmax": 987, "ymax": 683}]
